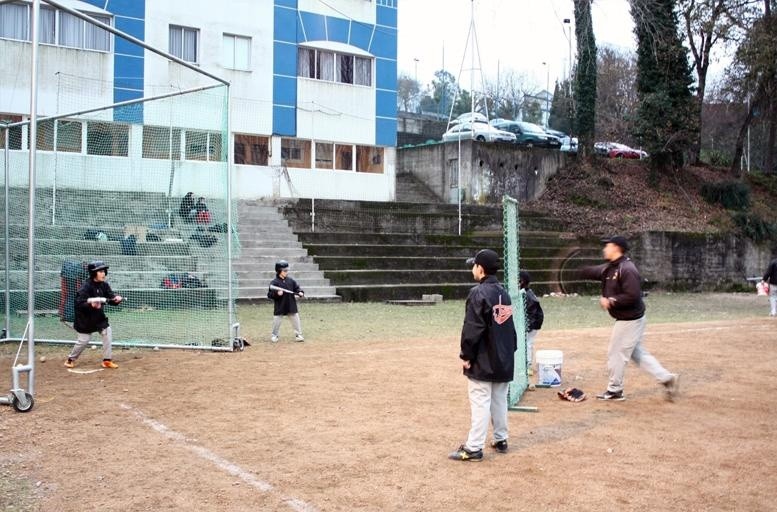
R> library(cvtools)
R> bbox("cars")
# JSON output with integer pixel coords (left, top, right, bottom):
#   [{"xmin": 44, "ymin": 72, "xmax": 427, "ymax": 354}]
[
  {"xmin": 592, "ymin": 141, "xmax": 648, "ymax": 159},
  {"xmin": 490, "ymin": 116, "xmax": 578, "ymax": 153},
  {"xmin": 441, "ymin": 123, "xmax": 516, "ymax": 145},
  {"xmin": 449, "ymin": 113, "xmax": 487, "ymax": 127}
]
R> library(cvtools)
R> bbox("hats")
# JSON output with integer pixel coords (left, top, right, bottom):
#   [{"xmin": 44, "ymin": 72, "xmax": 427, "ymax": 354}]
[
  {"xmin": 466, "ymin": 249, "xmax": 501, "ymax": 269},
  {"xmin": 600, "ymin": 235, "xmax": 628, "ymax": 250}
]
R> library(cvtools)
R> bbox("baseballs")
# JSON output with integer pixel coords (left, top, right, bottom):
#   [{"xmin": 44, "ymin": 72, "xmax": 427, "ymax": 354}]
[
  {"xmin": 39, "ymin": 356, "xmax": 45, "ymax": 362},
  {"xmin": 91, "ymin": 345, "xmax": 96, "ymax": 349}
]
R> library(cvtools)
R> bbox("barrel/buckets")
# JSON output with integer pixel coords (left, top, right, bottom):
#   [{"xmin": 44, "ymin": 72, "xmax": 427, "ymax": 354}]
[
  {"xmin": 755, "ymin": 282, "xmax": 770, "ymax": 297},
  {"xmin": 535, "ymin": 349, "xmax": 564, "ymax": 387}
]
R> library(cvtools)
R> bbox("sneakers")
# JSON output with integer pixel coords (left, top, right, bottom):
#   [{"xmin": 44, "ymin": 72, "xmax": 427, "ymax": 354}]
[
  {"xmin": 448, "ymin": 448, "xmax": 484, "ymax": 462},
  {"xmin": 296, "ymin": 335, "xmax": 304, "ymax": 342},
  {"xmin": 664, "ymin": 374, "xmax": 680, "ymax": 402},
  {"xmin": 491, "ymin": 440, "xmax": 507, "ymax": 452},
  {"xmin": 596, "ymin": 389, "xmax": 625, "ymax": 401},
  {"xmin": 101, "ymin": 360, "xmax": 118, "ymax": 368},
  {"xmin": 64, "ymin": 360, "xmax": 74, "ymax": 369},
  {"xmin": 272, "ymin": 336, "xmax": 278, "ymax": 343}
]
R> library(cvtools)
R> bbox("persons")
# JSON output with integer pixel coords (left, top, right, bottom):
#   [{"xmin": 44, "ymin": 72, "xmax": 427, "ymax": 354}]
[
  {"xmin": 760, "ymin": 258, "xmax": 776, "ymax": 317},
  {"xmin": 178, "ymin": 192, "xmax": 194, "ymax": 223},
  {"xmin": 194, "ymin": 197, "xmax": 208, "ymax": 223},
  {"xmin": 267, "ymin": 260, "xmax": 304, "ymax": 342},
  {"xmin": 62, "ymin": 259, "xmax": 123, "ymax": 368},
  {"xmin": 446, "ymin": 248, "xmax": 516, "ymax": 463},
  {"xmin": 518, "ymin": 270, "xmax": 544, "ymax": 376},
  {"xmin": 594, "ymin": 236, "xmax": 680, "ymax": 403}
]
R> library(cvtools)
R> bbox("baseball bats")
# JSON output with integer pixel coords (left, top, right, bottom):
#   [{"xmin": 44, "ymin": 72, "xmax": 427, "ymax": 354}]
[
  {"xmin": 270, "ymin": 284, "xmax": 304, "ymax": 298},
  {"xmin": 87, "ymin": 296, "xmax": 129, "ymax": 302}
]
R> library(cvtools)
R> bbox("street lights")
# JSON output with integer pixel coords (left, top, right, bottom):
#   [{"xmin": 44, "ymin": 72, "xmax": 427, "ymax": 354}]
[
  {"xmin": 562, "ymin": 18, "xmax": 572, "ymax": 153},
  {"xmin": 413, "ymin": 58, "xmax": 419, "ymax": 120},
  {"xmin": 542, "ymin": 62, "xmax": 548, "ymax": 129}
]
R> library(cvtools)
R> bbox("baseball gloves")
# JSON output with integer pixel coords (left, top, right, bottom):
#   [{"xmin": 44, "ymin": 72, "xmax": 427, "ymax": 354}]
[{"xmin": 557, "ymin": 387, "xmax": 587, "ymax": 402}]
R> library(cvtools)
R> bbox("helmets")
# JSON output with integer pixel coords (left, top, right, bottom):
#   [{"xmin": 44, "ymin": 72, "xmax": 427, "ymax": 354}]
[
  {"xmin": 88, "ymin": 261, "xmax": 109, "ymax": 278},
  {"xmin": 275, "ymin": 261, "xmax": 288, "ymax": 272}
]
